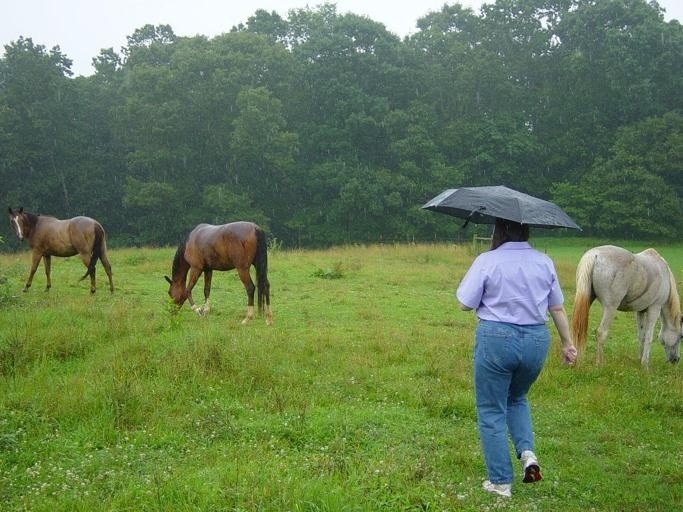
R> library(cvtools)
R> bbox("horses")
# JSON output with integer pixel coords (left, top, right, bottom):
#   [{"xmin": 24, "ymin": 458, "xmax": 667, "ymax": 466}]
[
  {"xmin": 7, "ymin": 206, "xmax": 115, "ymax": 296},
  {"xmin": 569, "ymin": 244, "xmax": 683, "ymax": 370},
  {"xmin": 164, "ymin": 221, "xmax": 273, "ymax": 327}
]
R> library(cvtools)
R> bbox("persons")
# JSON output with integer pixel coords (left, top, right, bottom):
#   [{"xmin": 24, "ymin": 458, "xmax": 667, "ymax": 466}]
[{"xmin": 455, "ymin": 219, "xmax": 577, "ymax": 496}]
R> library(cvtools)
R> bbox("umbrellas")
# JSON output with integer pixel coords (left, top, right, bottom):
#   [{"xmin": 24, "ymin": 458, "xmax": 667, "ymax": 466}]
[{"xmin": 419, "ymin": 186, "xmax": 583, "ymax": 229}]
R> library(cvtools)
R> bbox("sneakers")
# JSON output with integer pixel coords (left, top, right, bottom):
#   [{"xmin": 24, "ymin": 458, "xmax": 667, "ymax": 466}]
[
  {"xmin": 519, "ymin": 451, "xmax": 542, "ymax": 483},
  {"xmin": 482, "ymin": 480, "xmax": 511, "ymax": 498}
]
